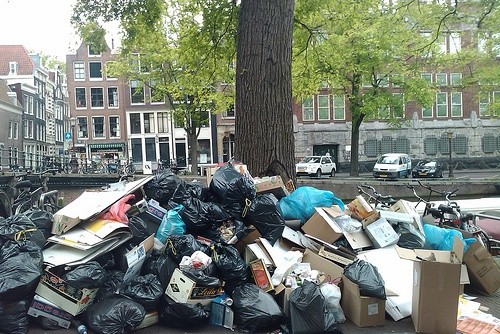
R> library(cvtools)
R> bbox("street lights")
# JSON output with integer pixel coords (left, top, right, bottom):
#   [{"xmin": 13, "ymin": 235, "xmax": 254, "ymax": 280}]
[
  {"xmin": 446, "ymin": 130, "xmax": 455, "ymax": 178},
  {"xmin": 224, "ymin": 130, "xmax": 231, "ymax": 162}
]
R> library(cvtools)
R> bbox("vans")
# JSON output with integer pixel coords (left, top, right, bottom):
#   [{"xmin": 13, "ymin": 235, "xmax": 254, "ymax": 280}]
[{"xmin": 373, "ymin": 153, "xmax": 412, "ymax": 179}]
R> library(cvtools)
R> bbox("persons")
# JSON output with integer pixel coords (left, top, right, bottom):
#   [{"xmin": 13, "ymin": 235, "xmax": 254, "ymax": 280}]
[
  {"xmin": 175, "ymin": 160, "xmax": 185, "ymax": 174},
  {"xmin": 160, "ymin": 159, "xmax": 166, "ymax": 169}
]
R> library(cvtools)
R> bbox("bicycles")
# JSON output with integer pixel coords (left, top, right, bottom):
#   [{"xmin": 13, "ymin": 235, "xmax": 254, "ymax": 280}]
[
  {"xmin": 357, "ymin": 179, "xmax": 491, "ymax": 253},
  {"xmin": 61, "ymin": 156, "xmax": 182, "ymax": 176},
  {"xmin": 14, "ymin": 168, "xmax": 58, "ymax": 216}
]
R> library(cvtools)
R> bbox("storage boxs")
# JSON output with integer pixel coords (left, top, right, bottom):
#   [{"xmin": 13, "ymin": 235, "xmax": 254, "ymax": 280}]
[
  {"xmin": 302, "ymin": 195, "xmax": 500, "ymax": 334},
  {"xmin": 255, "ymin": 177, "xmax": 290, "ymax": 199},
  {"xmin": 234, "ymin": 225, "xmax": 298, "ymax": 316},
  {"xmin": 128, "ymin": 199, "xmax": 147, "ymax": 218},
  {"xmin": 164, "ymin": 268, "xmax": 234, "ymax": 330},
  {"xmin": 35, "ymin": 266, "xmax": 98, "ymax": 317},
  {"xmin": 133, "ymin": 309, "xmax": 158, "ymax": 330},
  {"xmin": 147, "ymin": 199, "xmax": 167, "ymax": 221},
  {"xmin": 27, "ymin": 294, "xmax": 72, "ymax": 328}
]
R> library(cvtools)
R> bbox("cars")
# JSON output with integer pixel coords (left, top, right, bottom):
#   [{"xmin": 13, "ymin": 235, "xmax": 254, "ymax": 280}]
[
  {"xmin": 295, "ymin": 156, "xmax": 337, "ymax": 179},
  {"xmin": 411, "ymin": 158, "xmax": 444, "ymax": 180}
]
